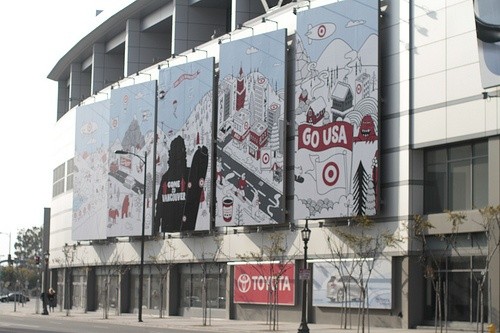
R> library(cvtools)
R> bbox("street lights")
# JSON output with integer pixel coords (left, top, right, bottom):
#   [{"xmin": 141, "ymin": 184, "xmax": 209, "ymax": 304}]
[
  {"xmin": 41, "ymin": 251, "xmax": 49, "ymax": 316},
  {"xmin": 297, "ymin": 219, "xmax": 311, "ymax": 333},
  {"xmin": 0, "ymin": 231, "xmax": 12, "ymax": 289},
  {"xmin": 114, "ymin": 150, "xmax": 148, "ymax": 322}
]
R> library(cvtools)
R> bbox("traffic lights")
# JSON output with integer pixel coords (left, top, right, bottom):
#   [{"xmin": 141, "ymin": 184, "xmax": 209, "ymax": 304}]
[{"xmin": 36, "ymin": 256, "xmax": 39, "ymax": 265}]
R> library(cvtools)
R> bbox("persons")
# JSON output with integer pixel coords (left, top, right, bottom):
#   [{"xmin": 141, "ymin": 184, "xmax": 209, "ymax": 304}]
[
  {"xmin": 48, "ymin": 288, "xmax": 56, "ymax": 313},
  {"xmin": 40, "ymin": 292, "xmax": 44, "ymax": 301}
]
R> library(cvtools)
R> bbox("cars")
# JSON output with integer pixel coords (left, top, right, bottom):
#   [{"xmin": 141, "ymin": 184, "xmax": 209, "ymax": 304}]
[{"xmin": 0, "ymin": 293, "xmax": 30, "ymax": 303}]
